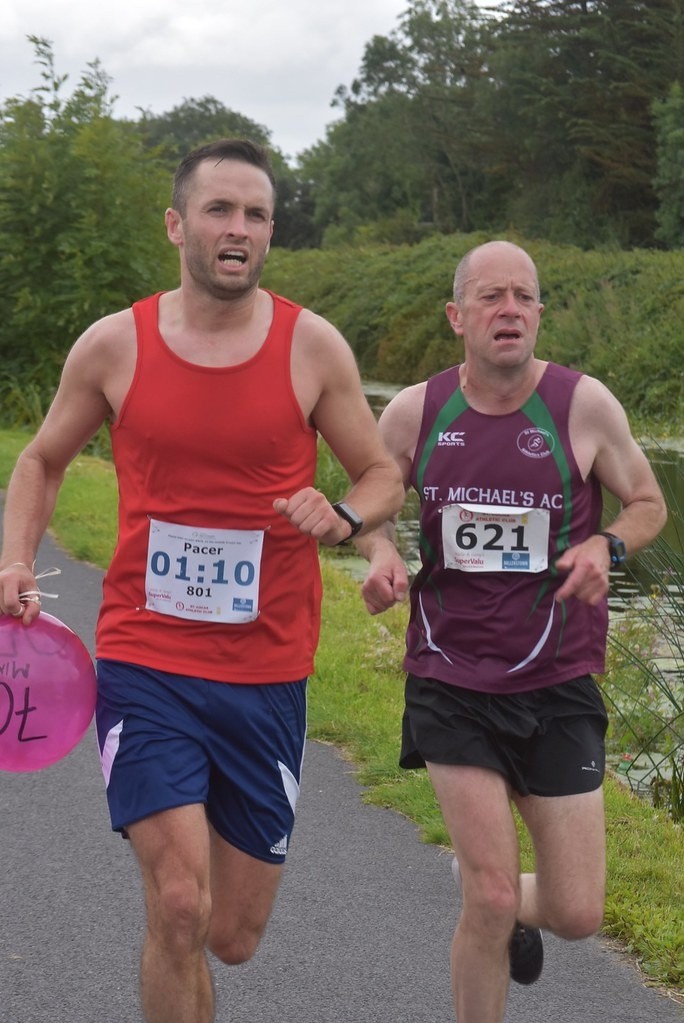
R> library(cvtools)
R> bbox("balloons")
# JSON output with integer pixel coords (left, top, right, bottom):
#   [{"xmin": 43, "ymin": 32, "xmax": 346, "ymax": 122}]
[{"xmin": 0, "ymin": 609, "xmax": 98, "ymax": 773}]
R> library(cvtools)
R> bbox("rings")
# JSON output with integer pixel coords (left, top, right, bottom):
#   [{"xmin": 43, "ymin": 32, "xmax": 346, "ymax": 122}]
[{"xmin": 13, "ymin": 607, "xmax": 22, "ymax": 616}]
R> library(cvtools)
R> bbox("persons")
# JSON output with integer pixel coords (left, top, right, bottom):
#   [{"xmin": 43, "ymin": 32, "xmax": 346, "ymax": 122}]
[
  {"xmin": 0, "ymin": 139, "xmax": 406, "ymax": 1023},
  {"xmin": 352, "ymin": 240, "xmax": 669, "ymax": 1023}
]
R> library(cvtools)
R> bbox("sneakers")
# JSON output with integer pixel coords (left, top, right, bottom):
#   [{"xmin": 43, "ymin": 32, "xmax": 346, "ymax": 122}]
[{"xmin": 453, "ymin": 856, "xmax": 544, "ymax": 986}]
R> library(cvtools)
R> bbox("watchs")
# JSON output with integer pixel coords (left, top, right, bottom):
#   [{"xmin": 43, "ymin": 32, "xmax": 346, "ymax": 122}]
[
  {"xmin": 331, "ymin": 500, "xmax": 363, "ymax": 548},
  {"xmin": 598, "ymin": 531, "xmax": 626, "ymax": 570}
]
